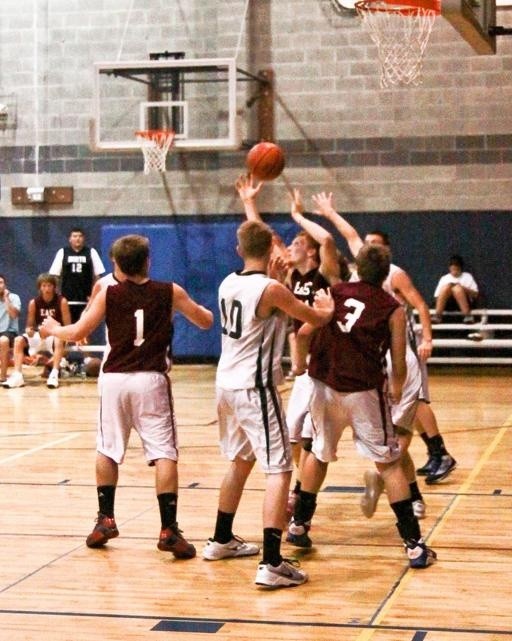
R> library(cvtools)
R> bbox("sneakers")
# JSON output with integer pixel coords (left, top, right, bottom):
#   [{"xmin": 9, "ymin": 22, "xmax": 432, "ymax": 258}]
[
  {"xmin": 203, "ymin": 538, "xmax": 259, "ymax": 561},
  {"xmin": 47, "ymin": 372, "xmax": 60, "ymax": 387},
  {"xmin": 254, "ymin": 558, "xmax": 307, "ymax": 587},
  {"xmin": 429, "ymin": 316, "xmax": 442, "ymax": 323},
  {"xmin": 158, "ymin": 521, "xmax": 196, "ymax": 558},
  {"xmin": 361, "ymin": 454, "xmax": 458, "ymax": 519},
  {"xmin": 463, "ymin": 316, "xmax": 475, "ymax": 323},
  {"xmin": 404, "ymin": 535, "xmax": 437, "ymax": 568},
  {"xmin": 2, "ymin": 371, "xmax": 26, "ymax": 388},
  {"xmin": 284, "ymin": 490, "xmax": 311, "ymax": 548},
  {"xmin": 87, "ymin": 511, "xmax": 118, "ymax": 547}
]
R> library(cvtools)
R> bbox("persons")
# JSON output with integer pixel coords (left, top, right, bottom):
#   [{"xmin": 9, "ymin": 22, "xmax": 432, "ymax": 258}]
[
  {"xmin": 75, "ymin": 241, "xmax": 127, "ymax": 350},
  {"xmin": 199, "ymin": 171, "xmax": 458, "ymax": 591},
  {"xmin": 0, "ymin": 273, "xmax": 74, "ymax": 390},
  {"xmin": 39, "ymin": 234, "xmax": 214, "ymax": 561},
  {"xmin": 49, "ymin": 227, "xmax": 105, "ymax": 371},
  {"xmin": 430, "ymin": 255, "xmax": 482, "ymax": 324},
  {"xmin": 0, "ymin": 276, "xmax": 24, "ymax": 384}
]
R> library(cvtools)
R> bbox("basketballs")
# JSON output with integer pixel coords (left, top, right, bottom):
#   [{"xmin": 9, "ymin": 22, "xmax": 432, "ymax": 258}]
[{"xmin": 246, "ymin": 142, "xmax": 284, "ymax": 181}]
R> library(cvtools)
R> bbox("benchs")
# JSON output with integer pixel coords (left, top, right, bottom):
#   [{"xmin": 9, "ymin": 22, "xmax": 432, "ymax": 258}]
[
  {"xmin": 413, "ymin": 308, "xmax": 512, "ymax": 365},
  {"xmin": 7, "ymin": 345, "xmax": 108, "ymax": 352}
]
